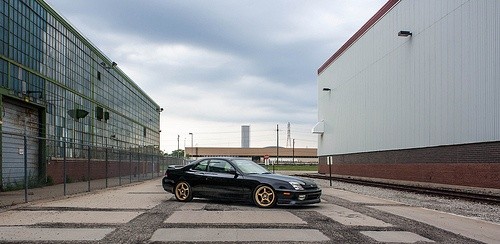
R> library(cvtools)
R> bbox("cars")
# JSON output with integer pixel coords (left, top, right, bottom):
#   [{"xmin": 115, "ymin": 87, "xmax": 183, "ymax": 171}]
[{"xmin": 161, "ymin": 155, "xmax": 323, "ymax": 207}]
